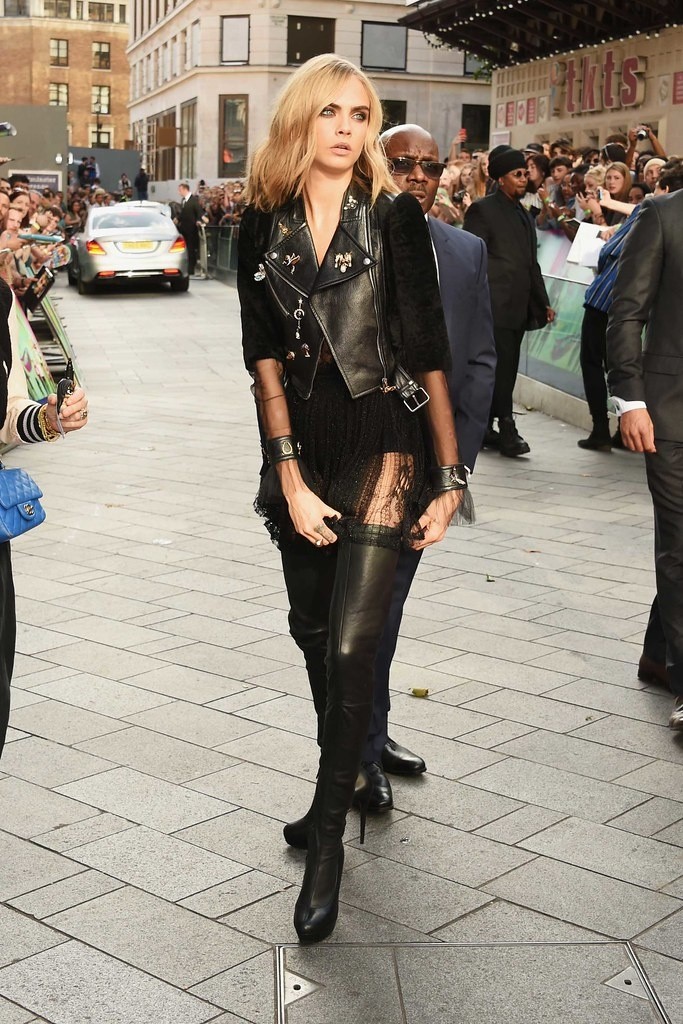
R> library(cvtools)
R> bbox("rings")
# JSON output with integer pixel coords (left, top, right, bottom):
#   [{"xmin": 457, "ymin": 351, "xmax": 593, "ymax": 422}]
[
  {"xmin": 80, "ymin": 410, "xmax": 88, "ymax": 420},
  {"xmin": 314, "ymin": 524, "xmax": 326, "ymax": 533},
  {"xmin": 315, "ymin": 538, "xmax": 324, "ymax": 546}
]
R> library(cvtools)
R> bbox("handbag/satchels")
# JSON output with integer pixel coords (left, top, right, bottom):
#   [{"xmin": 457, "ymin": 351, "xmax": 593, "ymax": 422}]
[{"xmin": 0, "ymin": 467, "xmax": 46, "ymax": 541}]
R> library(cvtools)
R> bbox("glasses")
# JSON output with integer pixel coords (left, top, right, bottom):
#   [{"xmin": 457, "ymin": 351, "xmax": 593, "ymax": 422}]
[
  {"xmin": 381, "ymin": 157, "xmax": 448, "ymax": 179},
  {"xmin": 503, "ymin": 169, "xmax": 531, "ymax": 178},
  {"xmin": 472, "ymin": 155, "xmax": 478, "ymax": 159},
  {"xmin": 584, "ymin": 158, "xmax": 599, "ymax": 163}
]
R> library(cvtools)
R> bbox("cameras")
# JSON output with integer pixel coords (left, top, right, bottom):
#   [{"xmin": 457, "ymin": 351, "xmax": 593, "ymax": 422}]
[
  {"xmin": 200, "ymin": 180, "xmax": 204, "ymax": 185},
  {"xmin": 636, "ymin": 129, "xmax": 647, "ymax": 141},
  {"xmin": 452, "ymin": 190, "xmax": 467, "ymax": 203}
]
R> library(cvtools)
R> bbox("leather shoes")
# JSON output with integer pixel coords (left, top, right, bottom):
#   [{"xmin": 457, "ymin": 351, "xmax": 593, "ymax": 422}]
[
  {"xmin": 637, "ymin": 654, "xmax": 680, "ymax": 695},
  {"xmin": 373, "ymin": 734, "xmax": 425, "ymax": 778},
  {"xmin": 669, "ymin": 693, "xmax": 683, "ymax": 731},
  {"xmin": 348, "ymin": 759, "xmax": 394, "ymax": 813}
]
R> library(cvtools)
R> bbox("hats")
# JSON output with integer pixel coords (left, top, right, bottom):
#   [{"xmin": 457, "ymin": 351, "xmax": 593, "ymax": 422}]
[
  {"xmin": 523, "ymin": 143, "xmax": 544, "ymax": 155},
  {"xmin": 487, "ymin": 144, "xmax": 528, "ymax": 180},
  {"xmin": 643, "ymin": 158, "xmax": 667, "ymax": 182}
]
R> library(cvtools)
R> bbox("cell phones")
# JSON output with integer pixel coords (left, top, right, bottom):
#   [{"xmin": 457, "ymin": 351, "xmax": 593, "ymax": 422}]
[
  {"xmin": 460, "ymin": 129, "xmax": 466, "ymax": 142},
  {"xmin": 598, "ymin": 189, "xmax": 602, "ymax": 200}
]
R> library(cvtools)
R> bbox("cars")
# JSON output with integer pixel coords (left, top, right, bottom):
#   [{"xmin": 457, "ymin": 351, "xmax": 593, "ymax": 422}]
[
  {"xmin": 65, "ymin": 204, "xmax": 190, "ymax": 295},
  {"xmin": 115, "ymin": 198, "xmax": 173, "ymax": 219}
]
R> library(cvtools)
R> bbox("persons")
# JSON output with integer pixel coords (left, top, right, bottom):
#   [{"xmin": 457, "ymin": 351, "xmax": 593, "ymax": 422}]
[
  {"xmin": 604, "ymin": 187, "xmax": 683, "ymax": 731},
  {"xmin": 87, "ymin": 155, "xmax": 149, "ymax": 207},
  {"xmin": 461, "ymin": 146, "xmax": 556, "ymax": 456},
  {"xmin": 578, "ymin": 157, "xmax": 683, "ymax": 449},
  {"xmin": 167, "ymin": 178, "xmax": 250, "ymax": 277},
  {"xmin": 0, "ymin": 173, "xmax": 87, "ymax": 294},
  {"xmin": 0, "ymin": 279, "xmax": 91, "ymax": 758},
  {"xmin": 235, "ymin": 53, "xmax": 477, "ymax": 943},
  {"xmin": 427, "ymin": 124, "xmax": 671, "ymax": 245},
  {"xmin": 355, "ymin": 126, "xmax": 497, "ymax": 823}
]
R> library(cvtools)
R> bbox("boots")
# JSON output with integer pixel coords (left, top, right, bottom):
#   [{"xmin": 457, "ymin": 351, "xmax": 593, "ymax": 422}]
[
  {"xmin": 267, "ymin": 535, "xmax": 369, "ymax": 851},
  {"xmin": 481, "ymin": 423, "xmax": 501, "ymax": 451},
  {"xmin": 610, "ymin": 415, "xmax": 630, "ymax": 450},
  {"xmin": 292, "ymin": 523, "xmax": 425, "ymax": 946},
  {"xmin": 578, "ymin": 418, "xmax": 612, "ymax": 451},
  {"xmin": 497, "ymin": 419, "xmax": 530, "ymax": 457}
]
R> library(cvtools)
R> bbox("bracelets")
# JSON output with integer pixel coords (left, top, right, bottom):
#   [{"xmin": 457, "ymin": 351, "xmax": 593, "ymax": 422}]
[
  {"xmin": 444, "ymin": 200, "xmax": 451, "ymax": 206},
  {"xmin": 21, "ymin": 278, "xmax": 25, "ymax": 287},
  {"xmin": 525, "ymin": 204, "xmax": 531, "ymax": 209},
  {"xmin": 267, "ymin": 435, "xmax": 300, "ymax": 466},
  {"xmin": 38, "ymin": 405, "xmax": 60, "ymax": 443},
  {"xmin": 543, "ymin": 196, "xmax": 550, "ymax": 203},
  {"xmin": 434, "ymin": 464, "xmax": 471, "ymax": 492},
  {"xmin": 557, "ymin": 214, "xmax": 565, "ymax": 221},
  {"xmin": 584, "ymin": 208, "xmax": 592, "ymax": 214}
]
